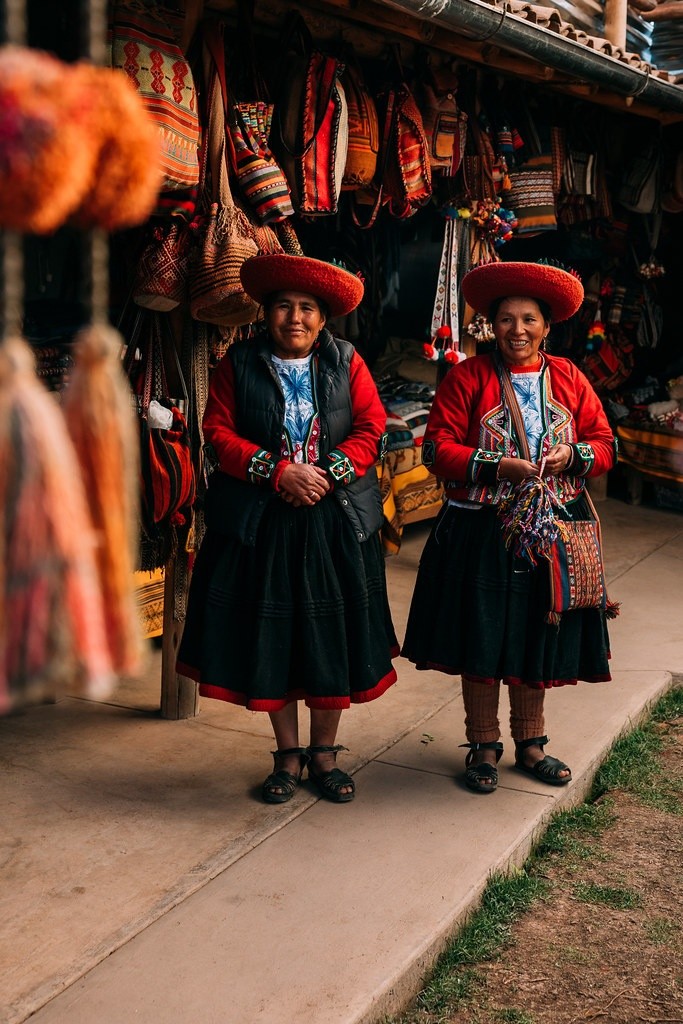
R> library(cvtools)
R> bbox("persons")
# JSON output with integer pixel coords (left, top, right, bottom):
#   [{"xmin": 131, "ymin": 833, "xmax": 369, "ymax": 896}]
[
  {"xmin": 401, "ymin": 263, "xmax": 619, "ymax": 792},
  {"xmin": 174, "ymin": 255, "xmax": 400, "ymax": 804}
]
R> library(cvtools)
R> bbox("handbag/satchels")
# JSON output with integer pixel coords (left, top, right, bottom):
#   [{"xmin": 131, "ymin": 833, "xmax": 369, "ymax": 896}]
[
  {"xmin": 545, "ymin": 520, "xmax": 623, "ymax": 626},
  {"xmin": 70, "ymin": 0, "xmax": 683, "ymax": 396},
  {"xmin": 117, "ymin": 327, "xmax": 197, "ymax": 573}
]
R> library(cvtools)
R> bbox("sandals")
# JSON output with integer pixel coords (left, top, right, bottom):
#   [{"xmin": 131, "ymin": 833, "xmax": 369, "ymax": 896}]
[
  {"xmin": 262, "ymin": 747, "xmax": 312, "ymax": 803},
  {"xmin": 514, "ymin": 735, "xmax": 572, "ymax": 785},
  {"xmin": 458, "ymin": 742, "xmax": 504, "ymax": 793},
  {"xmin": 307, "ymin": 744, "xmax": 356, "ymax": 801}
]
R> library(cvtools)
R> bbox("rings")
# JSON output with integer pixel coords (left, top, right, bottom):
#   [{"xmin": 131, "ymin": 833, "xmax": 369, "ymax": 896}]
[{"xmin": 310, "ymin": 492, "xmax": 316, "ymax": 497}]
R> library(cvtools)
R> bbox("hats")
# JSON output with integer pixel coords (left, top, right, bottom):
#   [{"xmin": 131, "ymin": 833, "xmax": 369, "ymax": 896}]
[
  {"xmin": 240, "ymin": 248, "xmax": 366, "ymax": 319},
  {"xmin": 460, "ymin": 258, "xmax": 585, "ymax": 324}
]
